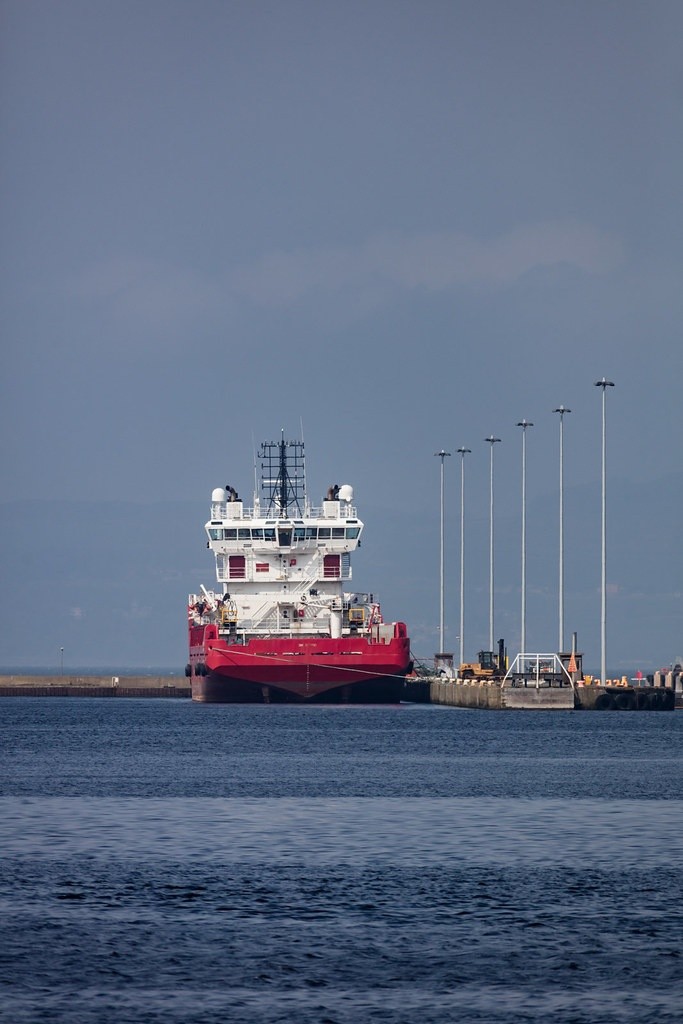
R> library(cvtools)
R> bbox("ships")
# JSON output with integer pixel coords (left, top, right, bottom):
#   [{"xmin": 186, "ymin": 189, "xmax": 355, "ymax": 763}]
[{"xmin": 184, "ymin": 416, "xmax": 415, "ymax": 706}]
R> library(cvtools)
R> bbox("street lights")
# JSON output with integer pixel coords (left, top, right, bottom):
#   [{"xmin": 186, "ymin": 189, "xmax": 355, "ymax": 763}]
[{"xmin": 434, "ymin": 376, "xmax": 619, "ymax": 688}]
